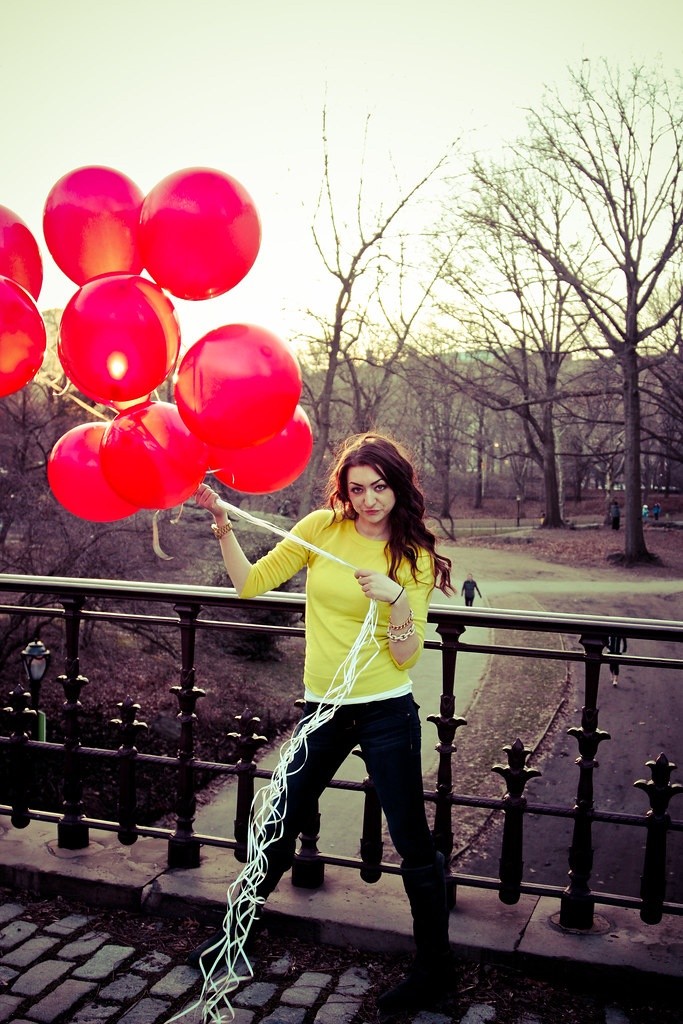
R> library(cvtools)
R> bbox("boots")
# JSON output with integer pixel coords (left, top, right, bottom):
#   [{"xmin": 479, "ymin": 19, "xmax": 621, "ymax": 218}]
[
  {"xmin": 187, "ymin": 834, "xmax": 296, "ymax": 969},
  {"xmin": 375, "ymin": 854, "xmax": 453, "ymax": 1013}
]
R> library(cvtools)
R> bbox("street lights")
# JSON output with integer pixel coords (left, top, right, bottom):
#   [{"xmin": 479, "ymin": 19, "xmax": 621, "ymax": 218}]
[{"xmin": 516, "ymin": 494, "xmax": 521, "ymax": 528}]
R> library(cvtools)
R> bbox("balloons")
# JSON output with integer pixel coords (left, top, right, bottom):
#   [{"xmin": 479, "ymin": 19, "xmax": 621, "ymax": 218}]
[
  {"xmin": 0, "ymin": 205, "xmax": 47, "ymax": 399},
  {"xmin": 140, "ymin": 167, "xmax": 261, "ymax": 300},
  {"xmin": 208, "ymin": 404, "xmax": 313, "ymax": 494},
  {"xmin": 57, "ymin": 271, "xmax": 181, "ymax": 400},
  {"xmin": 99, "ymin": 400, "xmax": 209, "ymax": 510},
  {"xmin": 58, "ymin": 342, "xmax": 152, "ymax": 413},
  {"xmin": 43, "ymin": 165, "xmax": 146, "ymax": 286},
  {"xmin": 176, "ymin": 323, "xmax": 302, "ymax": 451},
  {"xmin": 49, "ymin": 420, "xmax": 142, "ymax": 523}
]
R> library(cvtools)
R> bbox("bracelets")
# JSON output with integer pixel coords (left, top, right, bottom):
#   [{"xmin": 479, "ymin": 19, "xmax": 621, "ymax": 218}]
[
  {"xmin": 389, "ymin": 608, "xmax": 413, "ymax": 630},
  {"xmin": 211, "ymin": 521, "xmax": 234, "ymax": 540},
  {"xmin": 390, "ymin": 587, "xmax": 406, "ymax": 606},
  {"xmin": 387, "ymin": 625, "xmax": 416, "ymax": 643}
]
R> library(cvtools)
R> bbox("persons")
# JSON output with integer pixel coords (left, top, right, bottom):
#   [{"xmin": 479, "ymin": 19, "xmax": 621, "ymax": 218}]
[
  {"xmin": 188, "ymin": 429, "xmax": 461, "ymax": 1013},
  {"xmin": 642, "ymin": 504, "xmax": 649, "ymax": 519},
  {"xmin": 611, "ymin": 501, "xmax": 620, "ymax": 531},
  {"xmin": 461, "ymin": 573, "xmax": 482, "ymax": 607},
  {"xmin": 540, "ymin": 513, "xmax": 545, "ymax": 526},
  {"xmin": 651, "ymin": 503, "xmax": 661, "ymax": 521}
]
